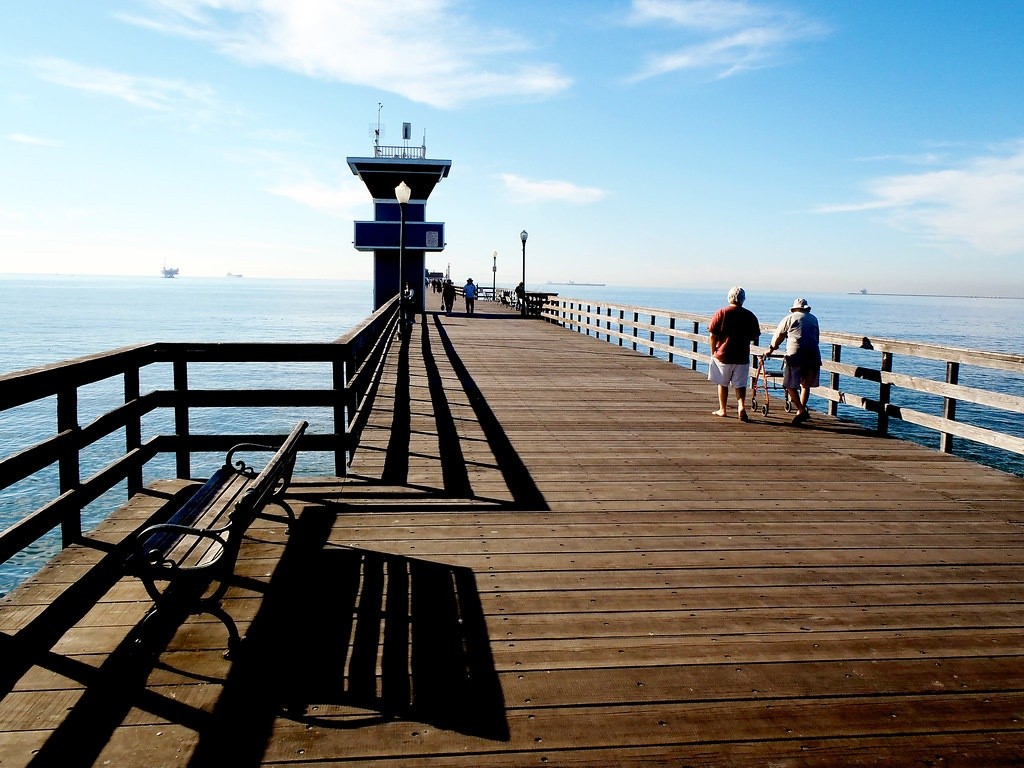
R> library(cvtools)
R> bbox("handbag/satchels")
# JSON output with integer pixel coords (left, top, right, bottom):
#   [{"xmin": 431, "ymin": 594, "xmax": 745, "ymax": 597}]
[{"xmin": 441, "ymin": 303, "xmax": 444, "ymax": 311}]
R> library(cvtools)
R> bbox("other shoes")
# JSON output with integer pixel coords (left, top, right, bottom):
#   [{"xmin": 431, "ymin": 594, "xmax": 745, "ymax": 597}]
[{"xmin": 792, "ymin": 410, "xmax": 808, "ymax": 424}]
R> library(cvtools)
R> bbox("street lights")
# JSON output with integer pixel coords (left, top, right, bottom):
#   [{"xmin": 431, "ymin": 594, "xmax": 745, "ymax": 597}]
[
  {"xmin": 394, "ymin": 182, "xmax": 411, "ymax": 341},
  {"xmin": 493, "ymin": 251, "xmax": 497, "ymax": 300},
  {"xmin": 520, "ymin": 230, "xmax": 528, "ymax": 315}
]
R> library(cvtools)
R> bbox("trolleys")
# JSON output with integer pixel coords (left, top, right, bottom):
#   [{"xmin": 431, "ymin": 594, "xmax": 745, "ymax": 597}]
[{"xmin": 751, "ymin": 354, "xmax": 792, "ymax": 417}]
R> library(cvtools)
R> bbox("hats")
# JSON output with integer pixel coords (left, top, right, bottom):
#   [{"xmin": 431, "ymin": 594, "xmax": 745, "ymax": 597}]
[
  {"xmin": 790, "ymin": 298, "xmax": 811, "ymax": 313},
  {"xmin": 467, "ymin": 278, "xmax": 473, "ymax": 282}
]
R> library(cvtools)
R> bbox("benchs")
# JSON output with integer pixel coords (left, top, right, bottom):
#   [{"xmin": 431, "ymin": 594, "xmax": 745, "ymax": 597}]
[
  {"xmin": 499, "ymin": 295, "xmax": 515, "ymax": 310},
  {"xmin": 122, "ymin": 420, "xmax": 310, "ymax": 659}
]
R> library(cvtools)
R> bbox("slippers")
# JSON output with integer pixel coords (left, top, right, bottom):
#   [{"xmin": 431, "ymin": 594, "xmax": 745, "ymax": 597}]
[
  {"xmin": 739, "ymin": 413, "xmax": 748, "ymax": 421},
  {"xmin": 711, "ymin": 411, "xmax": 727, "ymax": 417}
]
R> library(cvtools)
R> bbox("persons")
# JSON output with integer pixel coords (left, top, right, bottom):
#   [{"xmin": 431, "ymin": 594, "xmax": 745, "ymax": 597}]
[
  {"xmin": 764, "ymin": 297, "xmax": 822, "ymax": 425},
  {"xmin": 425, "ymin": 277, "xmax": 476, "ymax": 316},
  {"xmin": 515, "ymin": 281, "xmax": 525, "ymax": 312},
  {"xmin": 403, "ymin": 282, "xmax": 417, "ymax": 325},
  {"xmin": 707, "ymin": 287, "xmax": 762, "ymax": 421}
]
